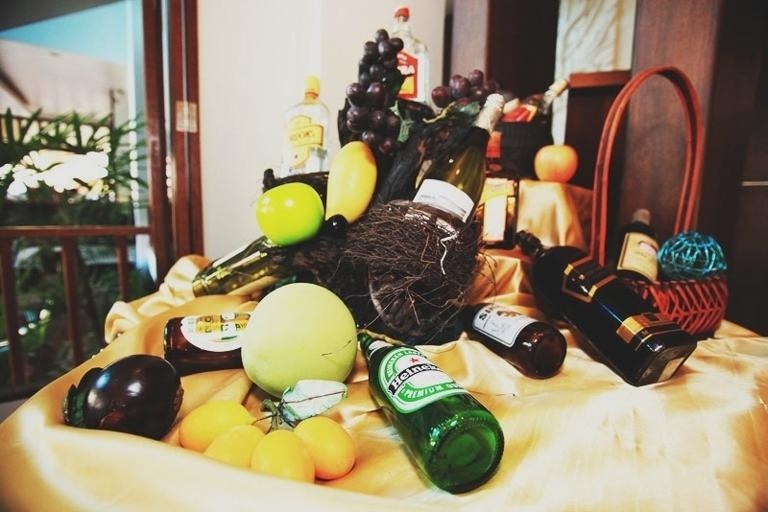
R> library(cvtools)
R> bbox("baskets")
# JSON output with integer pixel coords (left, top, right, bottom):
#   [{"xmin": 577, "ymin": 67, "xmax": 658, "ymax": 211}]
[{"xmin": 588, "ymin": 63, "xmax": 728, "ymax": 343}]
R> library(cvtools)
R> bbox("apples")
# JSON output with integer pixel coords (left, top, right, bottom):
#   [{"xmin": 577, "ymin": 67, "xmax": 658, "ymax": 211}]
[
  {"xmin": 533, "ymin": 145, "xmax": 579, "ymax": 182},
  {"xmin": 256, "ymin": 182, "xmax": 324, "ymax": 247}
]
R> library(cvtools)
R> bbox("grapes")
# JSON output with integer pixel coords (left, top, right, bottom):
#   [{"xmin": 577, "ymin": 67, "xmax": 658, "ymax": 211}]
[
  {"xmin": 337, "ymin": 28, "xmax": 404, "ymax": 154},
  {"xmin": 432, "ymin": 70, "xmax": 514, "ymax": 107}
]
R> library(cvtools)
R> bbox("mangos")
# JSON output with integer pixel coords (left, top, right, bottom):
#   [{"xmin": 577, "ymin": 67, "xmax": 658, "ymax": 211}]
[{"xmin": 325, "ymin": 141, "xmax": 377, "ymax": 224}]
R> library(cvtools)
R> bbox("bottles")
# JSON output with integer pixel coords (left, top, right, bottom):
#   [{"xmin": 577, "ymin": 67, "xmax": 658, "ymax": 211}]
[
  {"xmin": 404, "ymin": 92, "xmax": 507, "ymax": 233},
  {"xmin": 286, "ymin": 76, "xmax": 331, "ymax": 175},
  {"xmin": 457, "ymin": 302, "xmax": 568, "ymax": 380},
  {"xmin": 190, "ymin": 215, "xmax": 350, "ymax": 298},
  {"xmin": 389, "ymin": 8, "xmax": 432, "ymax": 107},
  {"xmin": 357, "ymin": 330, "xmax": 506, "ymax": 494},
  {"xmin": 502, "ymin": 78, "xmax": 571, "ymax": 123},
  {"xmin": 161, "ymin": 314, "xmax": 253, "ymax": 377},
  {"xmin": 512, "ymin": 209, "xmax": 698, "ymax": 387}
]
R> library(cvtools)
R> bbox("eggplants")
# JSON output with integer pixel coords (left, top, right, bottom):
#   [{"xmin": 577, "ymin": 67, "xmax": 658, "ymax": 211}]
[{"xmin": 62, "ymin": 353, "xmax": 184, "ymax": 439}]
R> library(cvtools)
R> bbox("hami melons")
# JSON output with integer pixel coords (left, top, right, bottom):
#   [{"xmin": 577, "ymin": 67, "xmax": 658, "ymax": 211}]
[{"xmin": 241, "ymin": 283, "xmax": 358, "ymax": 399}]
